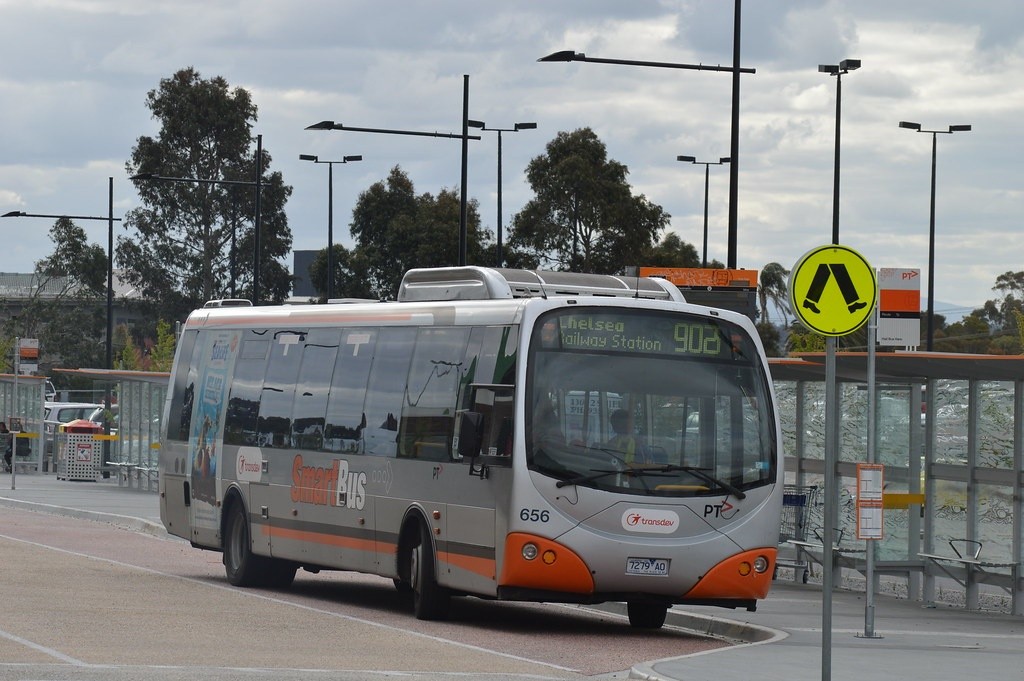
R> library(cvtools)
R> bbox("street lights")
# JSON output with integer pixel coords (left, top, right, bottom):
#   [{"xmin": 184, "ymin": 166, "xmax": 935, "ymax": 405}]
[
  {"xmin": 818, "ymin": 59, "xmax": 862, "ymax": 244},
  {"xmin": 299, "ymin": 154, "xmax": 362, "ymax": 298},
  {"xmin": 899, "ymin": 121, "xmax": 972, "ymax": 351},
  {"xmin": 677, "ymin": 156, "xmax": 731, "ymax": 269},
  {"xmin": 535, "ymin": 0, "xmax": 756, "ymax": 270},
  {"xmin": 305, "ymin": 75, "xmax": 481, "ymax": 267},
  {"xmin": 466, "ymin": 120, "xmax": 537, "ymax": 267},
  {"xmin": 127, "ymin": 135, "xmax": 271, "ymax": 306},
  {"xmin": 0, "ymin": 176, "xmax": 122, "ymax": 478}
]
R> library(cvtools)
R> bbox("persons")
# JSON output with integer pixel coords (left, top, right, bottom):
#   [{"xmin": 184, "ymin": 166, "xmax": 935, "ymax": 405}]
[
  {"xmin": 0, "ymin": 422, "xmax": 31, "ymax": 473},
  {"xmin": 607, "ymin": 408, "xmax": 656, "ymax": 467}
]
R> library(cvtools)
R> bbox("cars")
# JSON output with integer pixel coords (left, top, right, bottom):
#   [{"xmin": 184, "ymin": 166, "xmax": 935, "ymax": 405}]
[{"xmin": 43, "ymin": 398, "xmax": 153, "ymax": 456}]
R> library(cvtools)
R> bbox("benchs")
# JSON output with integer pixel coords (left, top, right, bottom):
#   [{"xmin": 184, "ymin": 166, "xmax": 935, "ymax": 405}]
[
  {"xmin": 136, "ymin": 467, "xmax": 159, "ymax": 484},
  {"xmin": 919, "ymin": 551, "xmax": 1021, "ymax": 617},
  {"xmin": 106, "ymin": 462, "xmax": 139, "ymax": 479},
  {"xmin": 788, "ymin": 539, "xmax": 866, "ymax": 578}
]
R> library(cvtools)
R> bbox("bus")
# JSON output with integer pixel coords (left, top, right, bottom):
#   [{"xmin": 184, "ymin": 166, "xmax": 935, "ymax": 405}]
[{"xmin": 157, "ymin": 265, "xmax": 784, "ymax": 634}]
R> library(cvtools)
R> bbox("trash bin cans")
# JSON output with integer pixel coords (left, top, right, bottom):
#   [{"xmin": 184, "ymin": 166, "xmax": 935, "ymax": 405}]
[{"xmin": 54, "ymin": 419, "xmax": 104, "ymax": 483}]
